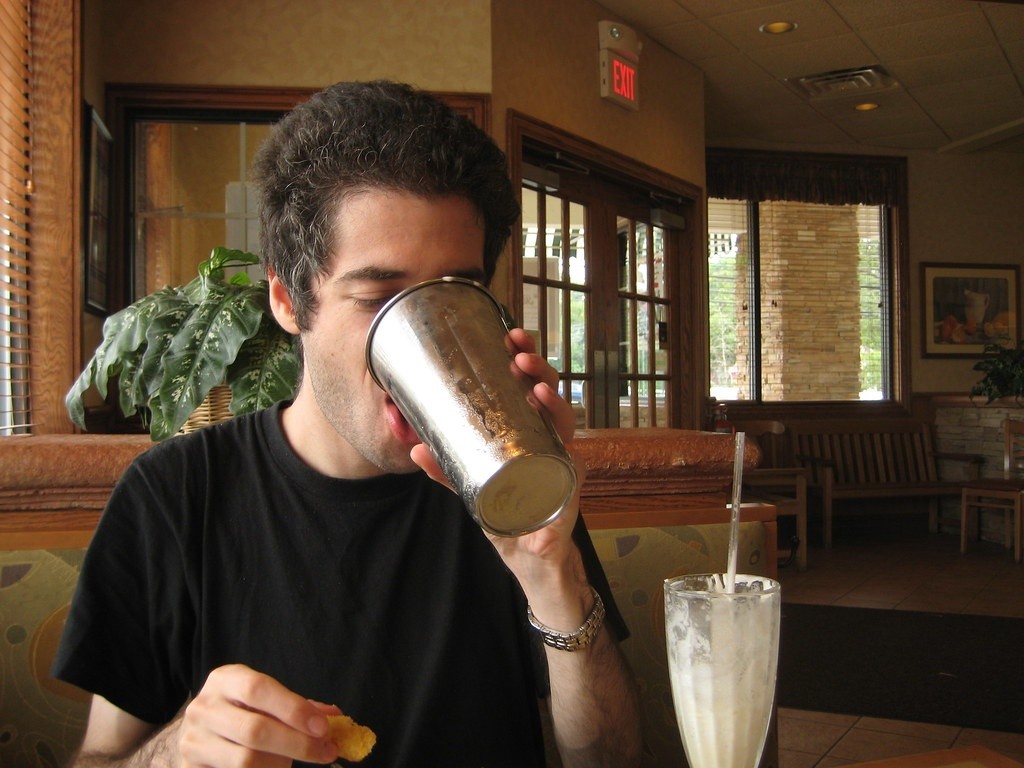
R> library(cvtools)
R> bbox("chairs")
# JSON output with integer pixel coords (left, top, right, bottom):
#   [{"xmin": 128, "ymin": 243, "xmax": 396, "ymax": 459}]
[
  {"xmin": 961, "ymin": 418, "xmax": 1024, "ymax": 563},
  {"xmin": 732, "ymin": 420, "xmax": 784, "ymax": 468}
]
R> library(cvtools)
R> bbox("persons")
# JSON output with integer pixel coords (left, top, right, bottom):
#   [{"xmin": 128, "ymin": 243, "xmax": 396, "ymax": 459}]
[{"xmin": 50, "ymin": 80, "xmax": 650, "ymax": 768}]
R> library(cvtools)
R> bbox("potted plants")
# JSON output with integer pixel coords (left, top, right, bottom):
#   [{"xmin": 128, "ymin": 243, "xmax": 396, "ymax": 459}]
[
  {"xmin": 969, "ymin": 334, "xmax": 1024, "ymax": 410},
  {"xmin": 64, "ymin": 246, "xmax": 300, "ymax": 441}
]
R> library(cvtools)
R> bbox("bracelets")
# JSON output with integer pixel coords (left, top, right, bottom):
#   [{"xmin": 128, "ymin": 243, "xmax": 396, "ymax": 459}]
[{"xmin": 526, "ymin": 585, "xmax": 606, "ymax": 651}]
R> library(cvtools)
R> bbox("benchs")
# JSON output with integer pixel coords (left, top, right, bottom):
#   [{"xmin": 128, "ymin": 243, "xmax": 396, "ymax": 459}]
[
  {"xmin": 771, "ymin": 416, "xmax": 985, "ymax": 550},
  {"xmin": 728, "ymin": 468, "xmax": 811, "ymax": 573}
]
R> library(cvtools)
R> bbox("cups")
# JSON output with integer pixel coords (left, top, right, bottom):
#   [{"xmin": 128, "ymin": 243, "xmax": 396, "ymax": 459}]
[
  {"xmin": 365, "ymin": 279, "xmax": 576, "ymax": 539},
  {"xmin": 663, "ymin": 573, "xmax": 782, "ymax": 768}
]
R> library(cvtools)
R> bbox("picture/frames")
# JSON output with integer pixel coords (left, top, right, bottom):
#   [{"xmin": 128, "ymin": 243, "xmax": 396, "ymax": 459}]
[
  {"xmin": 919, "ymin": 261, "xmax": 1023, "ymax": 357},
  {"xmin": 85, "ymin": 105, "xmax": 115, "ymax": 318}
]
